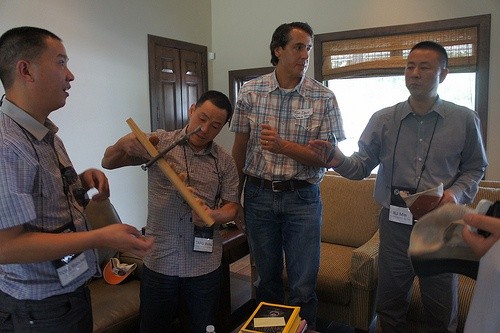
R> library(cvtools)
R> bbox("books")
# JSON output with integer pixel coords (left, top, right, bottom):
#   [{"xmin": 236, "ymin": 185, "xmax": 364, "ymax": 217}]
[{"xmin": 237, "ymin": 301, "xmax": 307, "ymax": 333}]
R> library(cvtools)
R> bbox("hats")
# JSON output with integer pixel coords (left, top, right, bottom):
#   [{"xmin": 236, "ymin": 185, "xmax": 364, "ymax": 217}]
[{"xmin": 103, "ymin": 258, "xmax": 137, "ymax": 285}]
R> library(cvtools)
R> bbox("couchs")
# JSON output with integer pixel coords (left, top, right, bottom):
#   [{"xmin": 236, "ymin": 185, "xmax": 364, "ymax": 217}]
[
  {"xmin": 374, "ymin": 178, "xmax": 500, "ymax": 333},
  {"xmin": 248, "ymin": 170, "xmax": 382, "ymax": 333},
  {"xmin": 83, "ymin": 194, "xmax": 144, "ymax": 333}
]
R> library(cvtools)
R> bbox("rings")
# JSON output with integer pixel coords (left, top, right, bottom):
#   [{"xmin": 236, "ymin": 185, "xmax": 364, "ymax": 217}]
[{"xmin": 265, "ymin": 140, "xmax": 269, "ymax": 145}]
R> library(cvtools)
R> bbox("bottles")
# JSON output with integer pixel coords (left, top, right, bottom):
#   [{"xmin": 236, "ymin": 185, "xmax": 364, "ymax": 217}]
[{"xmin": 206, "ymin": 325, "xmax": 216, "ymax": 333}]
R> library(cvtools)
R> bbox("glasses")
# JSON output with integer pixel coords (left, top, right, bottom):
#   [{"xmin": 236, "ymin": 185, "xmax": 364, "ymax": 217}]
[{"xmin": 61, "ymin": 166, "xmax": 90, "ymax": 210}]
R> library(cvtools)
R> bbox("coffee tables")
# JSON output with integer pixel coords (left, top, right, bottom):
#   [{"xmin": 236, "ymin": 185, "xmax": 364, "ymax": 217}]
[{"xmin": 231, "ymin": 321, "xmax": 322, "ymax": 333}]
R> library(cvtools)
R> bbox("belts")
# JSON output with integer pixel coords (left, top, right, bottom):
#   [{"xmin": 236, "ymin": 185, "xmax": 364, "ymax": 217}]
[{"xmin": 246, "ymin": 176, "xmax": 314, "ymax": 193}]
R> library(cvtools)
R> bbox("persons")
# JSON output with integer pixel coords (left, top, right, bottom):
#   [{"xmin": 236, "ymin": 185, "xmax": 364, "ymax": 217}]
[
  {"xmin": 308, "ymin": 40, "xmax": 489, "ymax": 333},
  {"xmin": 230, "ymin": 21, "xmax": 347, "ymax": 330},
  {"xmin": 462, "ymin": 199, "xmax": 500, "ymax": 333},
  {"xmin": 102, "ymin": 91, "xmax": 240, "ymax": 333},
  {"xmin": 0, "ymin": 26, "xmax": 156, "ymax": 333}
]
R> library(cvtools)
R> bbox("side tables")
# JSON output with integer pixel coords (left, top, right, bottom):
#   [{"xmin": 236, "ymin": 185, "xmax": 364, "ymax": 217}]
[{"xmin": 142, "ymin": 221, "xmax": 254, "ymax": 333}]
